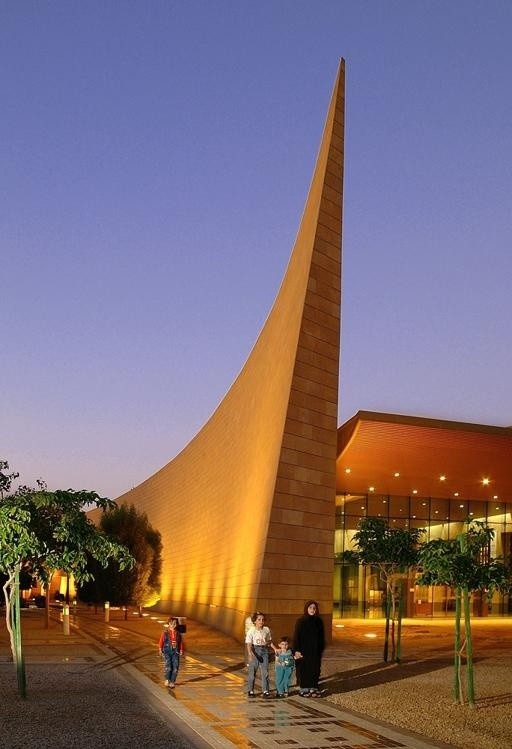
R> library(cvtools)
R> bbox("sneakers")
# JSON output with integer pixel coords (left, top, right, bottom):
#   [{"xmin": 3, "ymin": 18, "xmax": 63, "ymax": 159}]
[
  {"xmin": 247, "ymin": 688, "xmax": 322, "ymax": 698},
  {"xmin": 169, "ymin": 682, "xmax": 175, "ymax": 688},
  {"xmin": 164, "ymin": 680, "xmax": 169, "ymax": 686}
]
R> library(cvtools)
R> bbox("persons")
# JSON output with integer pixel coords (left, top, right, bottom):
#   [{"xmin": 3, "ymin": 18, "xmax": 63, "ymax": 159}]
[
  {"xmin": 158, "ymin": 615, "xmax": 185, "ymax": 689},
  {"xmin": 245, "ymin": 611, "xmax": 278, "ymax": 698},
  {"xmin": 273, "ymin": 635, "xmax": 295, "ymax": 698},
  {"xmin": 292, "ymin": 599, "xmax": 325, "ymax": 699}
]
